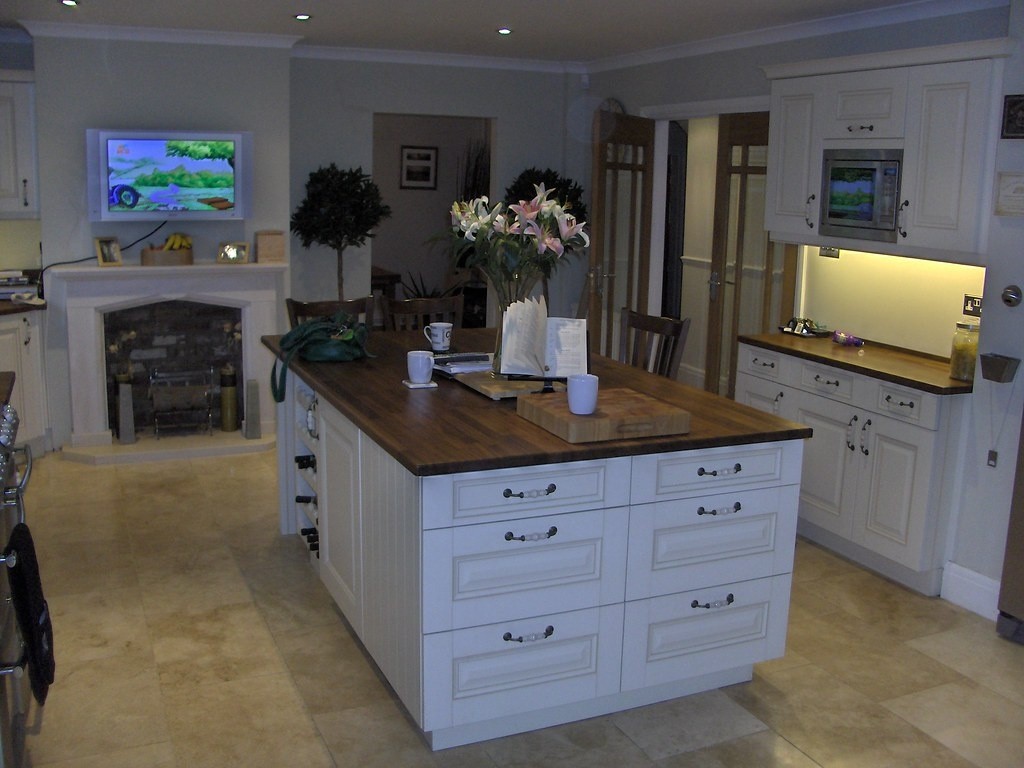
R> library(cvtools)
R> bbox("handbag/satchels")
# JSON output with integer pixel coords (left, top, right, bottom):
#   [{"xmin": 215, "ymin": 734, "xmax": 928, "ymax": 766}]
[{"xmin": 271, "ymin": 316, "xmax": 365, "ymax": 403}]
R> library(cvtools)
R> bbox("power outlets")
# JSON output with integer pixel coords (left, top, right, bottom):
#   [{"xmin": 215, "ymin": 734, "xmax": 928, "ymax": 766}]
[{"xmin": 962, "ymin": 293, "xmax": 983, "ymax": 316}]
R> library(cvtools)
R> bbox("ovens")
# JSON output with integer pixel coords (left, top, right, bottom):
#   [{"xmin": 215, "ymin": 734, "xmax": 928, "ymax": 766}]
[{"xmin": 818, "ymin": 148, "xmax": 903, "ymax": 243}]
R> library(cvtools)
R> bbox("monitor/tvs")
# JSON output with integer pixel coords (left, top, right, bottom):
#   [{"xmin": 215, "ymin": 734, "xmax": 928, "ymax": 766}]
[{"xmin": 85, "ymin": 129, "xmax": 252, "ymax": 221}]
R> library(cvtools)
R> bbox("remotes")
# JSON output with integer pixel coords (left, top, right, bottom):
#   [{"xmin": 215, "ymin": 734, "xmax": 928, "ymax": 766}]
[{"xmin": 435, "ymin": 352, "xmax": 489, "ymax": 364}]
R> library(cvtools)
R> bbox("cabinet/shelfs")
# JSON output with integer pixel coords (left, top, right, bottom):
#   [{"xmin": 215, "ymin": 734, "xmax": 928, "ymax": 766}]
[
  {"xmin": 0, "ymin": 309, "xmax": 47, "ymax": 464},
  {"xmin": 733, "ymin": 341, "xmax": 974, "ymax": 597},
  {"xmin": 763, "ymin": 55, "xmax": 1006, "ymax": 267},
  {"xmin": 0, "ymin": 68, "xmax": 41, "ymax": 221},
  {"xmin": 273, "ymin": 355, "xmax": 804, "ymax": 752}
]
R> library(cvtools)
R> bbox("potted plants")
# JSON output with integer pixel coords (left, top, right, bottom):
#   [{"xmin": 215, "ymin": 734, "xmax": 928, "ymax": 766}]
[{"xmin": 290, "ymin": 161, "xmax": 394, "ymax": 300}]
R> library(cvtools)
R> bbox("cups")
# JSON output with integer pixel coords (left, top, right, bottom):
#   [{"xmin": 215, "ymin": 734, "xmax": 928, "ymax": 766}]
[
  {"xmin": 424, "ymin": 322, "xmax": 453, "ymax": 350},
  {"xmin": 567, "ymin": 374, "xmax": 599, "ymax": 415},
  {"xmin": 407, "ymin": 350, "xmax": 434, "ymax": 384}
]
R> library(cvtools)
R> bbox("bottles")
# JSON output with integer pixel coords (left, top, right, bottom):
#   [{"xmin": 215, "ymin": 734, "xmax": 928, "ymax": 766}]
[{"xmin": 832, "ymin": 331, "xmax": 864, "ymax": 346}]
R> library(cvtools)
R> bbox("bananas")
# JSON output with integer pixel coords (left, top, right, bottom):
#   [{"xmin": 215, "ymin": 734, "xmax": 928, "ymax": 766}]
[{"xmin": 163, "ymin": 233, "xmax": 194, "ymax": 251}]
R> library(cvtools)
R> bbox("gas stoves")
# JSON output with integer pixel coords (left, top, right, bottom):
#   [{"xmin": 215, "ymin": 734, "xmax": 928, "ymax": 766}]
[{"xmin": 0, "ymin": 270, "xmax": 42, "ymax": 301}]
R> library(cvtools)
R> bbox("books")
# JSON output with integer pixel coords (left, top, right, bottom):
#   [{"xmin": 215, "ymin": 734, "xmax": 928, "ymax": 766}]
[
  {"xmin": 0, "ymin": 270, "xmax": 29, "ymax": 285},
  {"xmin": 500, "ymin": 294, "xmax": 588, "ymax": 378},
  {"xmin": 434, "ymin": 352, "xmax": 494, "ymax": 373}
]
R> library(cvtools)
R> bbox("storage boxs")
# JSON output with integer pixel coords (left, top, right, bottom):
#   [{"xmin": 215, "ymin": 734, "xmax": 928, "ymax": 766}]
[{"xmin": 141, "ymin": 246, "xmax": 194, "ymax": 266}]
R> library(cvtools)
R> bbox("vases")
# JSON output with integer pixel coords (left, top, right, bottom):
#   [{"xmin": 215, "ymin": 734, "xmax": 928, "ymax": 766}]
[
  {"xmin": 221, "ymin": 367, "xmax": 238, "ymax": 432},
  {"xmin": 480, "ymin": 266, "xmax": 544, "ymax": 379},
  {"xmin": 113, "ymin": 374, "xmax": 131, "ymax": 439}
]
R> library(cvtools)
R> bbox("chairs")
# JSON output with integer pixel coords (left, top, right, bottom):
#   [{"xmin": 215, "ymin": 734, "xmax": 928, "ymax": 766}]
[
  {"xmin": 618, "ymin": 301, "xmax": 690, "ymax": 380},
  {"xmin": 149, "ymin": 366, "xmax": 216, "ymax": 438},
  {"xmin": 286, "ymin": 293, "xmax": 465, "ymax": 331}
]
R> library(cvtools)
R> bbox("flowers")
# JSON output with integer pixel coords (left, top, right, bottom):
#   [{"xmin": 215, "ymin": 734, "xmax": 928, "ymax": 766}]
[
  {"xmin": 108, "ymin": 330, "xmax": 135, "ymax": 373},
  {"xmin": 220, "ymin": 322, "xmax": 242, "ymax": 366},
  {"xmin": 421, "ymin": 181, "xmax": 591, "ymax": 361}
]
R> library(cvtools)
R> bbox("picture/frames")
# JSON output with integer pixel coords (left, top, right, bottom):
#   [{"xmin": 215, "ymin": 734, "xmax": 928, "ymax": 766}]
[
  {"xmin": 400, "ymin": 145, "xmax": 438, "ymax": 190},
  {"xmin": 94, "ymin": 235, "xmax": 122, "ymax": 267},
  {"xmin": 217, "ymin": 241, "xmax": 250, "ymax": 264}
]
